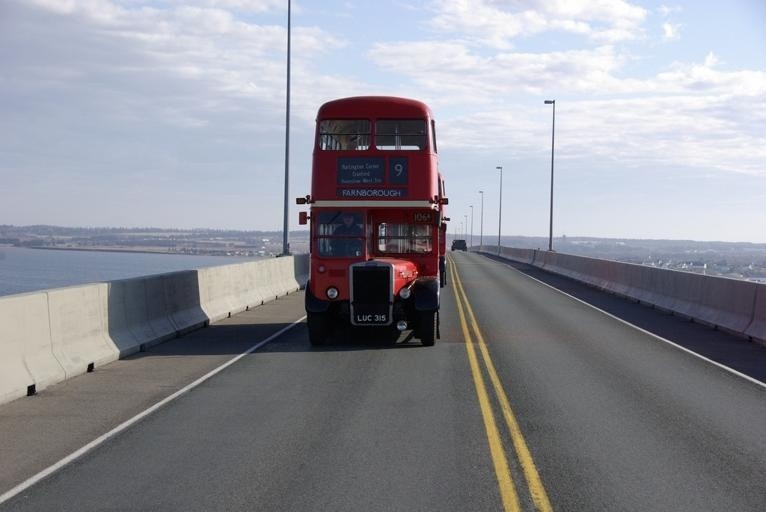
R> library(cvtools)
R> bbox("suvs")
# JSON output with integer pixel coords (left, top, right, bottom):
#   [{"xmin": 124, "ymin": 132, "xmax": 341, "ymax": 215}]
[{"xmin": 450, "ymin": 239, "xmax": 467, "ymax": 253}]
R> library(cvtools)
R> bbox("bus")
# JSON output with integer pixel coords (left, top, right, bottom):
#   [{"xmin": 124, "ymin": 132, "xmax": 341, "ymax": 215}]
[{"xmin": 292, "ymin": 93, "xmax": 447, "ymax": 351}]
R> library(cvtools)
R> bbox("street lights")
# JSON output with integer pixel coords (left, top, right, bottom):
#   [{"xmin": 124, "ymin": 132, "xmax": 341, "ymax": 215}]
[
  {"xmin": 543, "ymin": 98, "xmax": 557, "ymax": 251},
  {"xmin": 459, "ymin": 165, "xmax": 505, "ymax": 255}
]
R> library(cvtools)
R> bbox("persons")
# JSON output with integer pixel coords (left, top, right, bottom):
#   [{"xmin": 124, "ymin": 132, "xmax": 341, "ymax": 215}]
[{"xmin": 325, "ymin": 209, "xmax": 362, "ymax": 258}]
